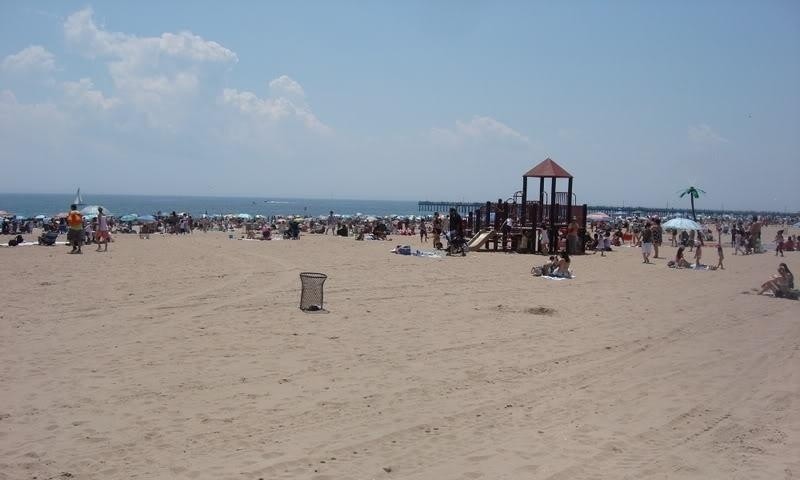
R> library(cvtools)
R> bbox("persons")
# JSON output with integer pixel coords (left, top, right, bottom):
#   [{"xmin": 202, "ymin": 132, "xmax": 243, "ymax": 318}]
[
  {"xmin": 68, "ymin": 204, "xmax": 110, "ymax": 253},
  {"xmin": 108, "ymin": 211, "xmax": 241, "ymax": 234},
  {"xmin": 246, "ymin": 210, "xmax": 428, "ymax": 242},
  {"xmin": 503, "ymin": 214, "xmax": 578, "ymax": 278},
  {"xmin": 430, "ymin": 208, "xmax": 463, "ymax": 249},
  {"xmin": 588, "ymin": 216, "xmax": 724, "ymax": 268},
  {"xmin": 751, "ymin": 263, "xmax": 794, "ymax": 296},
  {"xmin": 1, "ymin": 215, "xmax": 65, "ymax": 235},
  {"xmin": 721, "ymin": 217, "xmax": 800, "ymax": 256}
]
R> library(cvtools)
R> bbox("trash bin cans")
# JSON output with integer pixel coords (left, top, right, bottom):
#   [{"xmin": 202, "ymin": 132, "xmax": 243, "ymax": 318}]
[{"xmin": 300, "ymin": 272, "xmax": 327, "ymax": 311}]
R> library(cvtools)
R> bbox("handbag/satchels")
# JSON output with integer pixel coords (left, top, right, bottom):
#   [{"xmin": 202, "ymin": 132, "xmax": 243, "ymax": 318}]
[
  {"xmin": 67, "ymin": 215, "xmax": 82, "ymax": 225},
  {"xmin": 533, "ymin": 266, "xmax": 543, "ymax": 275},
  {"xmin": 784, "ymin": 288, "xmax": 799, "ymax": 299}
]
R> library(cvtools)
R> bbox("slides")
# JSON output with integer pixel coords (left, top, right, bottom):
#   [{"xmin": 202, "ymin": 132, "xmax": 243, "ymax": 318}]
[{"xmin": 467, "ymin": 229, "xmax": 496, "ymax": 250}]
